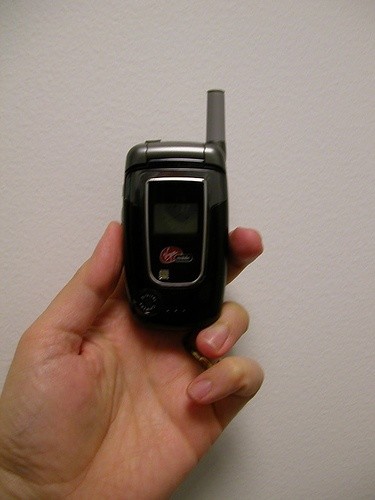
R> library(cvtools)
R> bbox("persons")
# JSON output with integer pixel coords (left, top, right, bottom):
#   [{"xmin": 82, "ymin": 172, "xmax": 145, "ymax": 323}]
[{"xmin": 1, "ymin": 219, "xmax": 265, "ymax": 499}]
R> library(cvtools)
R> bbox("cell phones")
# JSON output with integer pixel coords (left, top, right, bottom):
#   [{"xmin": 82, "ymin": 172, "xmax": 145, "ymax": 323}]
[{"xmin": 121, "ymin": 89, "xmax": 229, "ymax": 334}]
